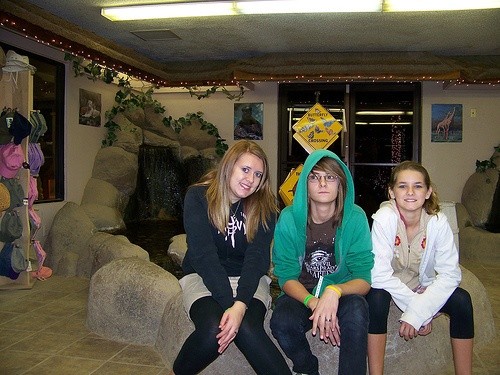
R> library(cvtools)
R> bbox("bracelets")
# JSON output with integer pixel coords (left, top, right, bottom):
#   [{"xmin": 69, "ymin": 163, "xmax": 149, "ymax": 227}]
[
  {"xmin": 322, "ymin": 285, "xmax": 341, "ymax": 298},
  {"xmin": 304, "ymin": 294, "xmax": 314, "ymax": 308}
]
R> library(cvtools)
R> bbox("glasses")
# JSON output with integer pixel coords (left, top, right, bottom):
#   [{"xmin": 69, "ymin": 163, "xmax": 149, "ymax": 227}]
[{"xmin": 307, "ymin": 173, "xmax": 340, "ymax": 183}]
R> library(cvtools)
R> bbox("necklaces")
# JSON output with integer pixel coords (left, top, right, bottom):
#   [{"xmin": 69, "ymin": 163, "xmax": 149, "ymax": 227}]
[{"xmin": 230, "ymin": 200, "xmax": 241, "ymax": 220}]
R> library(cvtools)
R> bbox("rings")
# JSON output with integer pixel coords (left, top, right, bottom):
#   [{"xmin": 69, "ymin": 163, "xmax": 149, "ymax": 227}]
[
  {"xmin": 234, "ymin": 331, "xmax": 237, "ymax": 335},
  {"xmin": 325, "ymin": 319, "xmax": 332, "ymax": 322}
]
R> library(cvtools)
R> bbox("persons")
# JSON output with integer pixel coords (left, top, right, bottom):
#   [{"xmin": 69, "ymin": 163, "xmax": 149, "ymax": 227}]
[
  {"xmin": 171, "ymin": 140, "xmax": 295, "ymax": 375},
  {"xmin": 367, "ymin": 161, "xmax": 475, "ymax": 375},
  {"xmin": 263, "ymin": 150, "xmax": 375, "ymax": 374}
]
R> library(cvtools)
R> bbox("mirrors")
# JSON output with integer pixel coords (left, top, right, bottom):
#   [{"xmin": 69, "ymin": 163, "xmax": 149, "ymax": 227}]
[{"xmin": 430, "ymin": 104, "xmax": 464, "ymax": 143}]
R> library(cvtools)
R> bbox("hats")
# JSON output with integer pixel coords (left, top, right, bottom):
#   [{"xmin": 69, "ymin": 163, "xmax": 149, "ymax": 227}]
[
  {"xmin": 0, "ymin": 107, "xmax": 32, "ymax": 280},
  {"xmin": 28, "ymin": 111, "xmax": 53, "ymax": 281}
]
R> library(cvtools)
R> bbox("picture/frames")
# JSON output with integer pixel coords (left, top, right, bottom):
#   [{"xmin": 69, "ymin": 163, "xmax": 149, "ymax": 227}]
[
  {"xmin": 234, "ymin": 102, "xmax": 263, "ymax": 141},
  {"xmin": 78, "ymin": 88, "xmax": 102, "ymax": 128}
]
